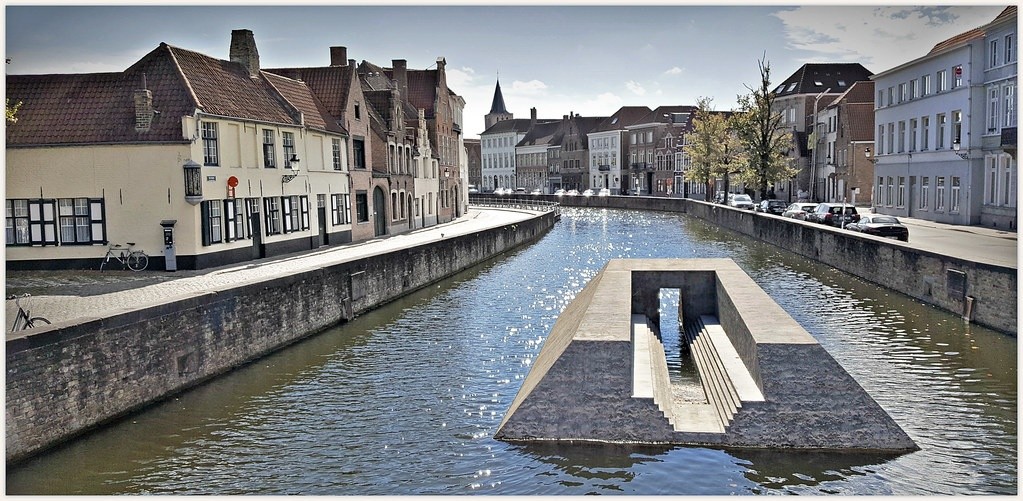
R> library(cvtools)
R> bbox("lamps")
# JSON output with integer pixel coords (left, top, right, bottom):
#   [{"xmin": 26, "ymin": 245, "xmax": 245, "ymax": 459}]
[
  {"xmin": 952, "ymin": 139, "xmax": 968, "ymax": 160},
  {"xmin": 864, "ymin": 147, "xmax": 879, "ymax": 164},
  {"xmin": 438, "ymin": 165, "xmax": 450, "ymax": 179},
  {"xmin": 282, "ymin": 154, "xmax": 301, "ymax": 183}
]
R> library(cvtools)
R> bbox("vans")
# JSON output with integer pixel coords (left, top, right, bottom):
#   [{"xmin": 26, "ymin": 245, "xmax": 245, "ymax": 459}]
[{"xmin": 467, "ymin": 184, "xmax": 479, "ymax": 194}]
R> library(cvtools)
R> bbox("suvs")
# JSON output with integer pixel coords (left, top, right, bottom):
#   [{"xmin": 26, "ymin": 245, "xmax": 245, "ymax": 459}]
[
  {"xmin": 728, "ymin": 193, "xmax": 756, "ymax": 211},
  {"xmin": 803, "ymin": 203, "xmax": 861, "ymax": 230}
]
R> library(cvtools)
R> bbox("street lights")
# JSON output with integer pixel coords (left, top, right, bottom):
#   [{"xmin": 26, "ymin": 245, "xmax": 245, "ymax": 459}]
[
  {"xmin": 439, "ymin": 168, "xmax": 450, "ymax": 184},
  {"xmin": 863, "ymin": 146, "xmax": 878, "ymax": 165},
  {"xmin": 282, "ymin": 154, "xmax": 301, "ymax": 185},
  {"xmin": 952, "ymin": 136, "xmax": 970, "ymax": 161}
]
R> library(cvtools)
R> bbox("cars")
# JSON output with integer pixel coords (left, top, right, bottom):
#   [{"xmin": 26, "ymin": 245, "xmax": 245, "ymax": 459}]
[
  {"xmin": 757, "ymin": 199, "xmax": 790, "ymax": 215},
  {"xmin": 714, "ymin": 190, "xmax": 731, "ymax": 202},
  {"xmin": 844, "ymin": 214, "xmax": 910, "ymax": 243},
  {"xmin": 782, "ymin": 201, "xmax": 820, "ymax": 222},
  {"xmin": 493, "ymin": 186, "xmax": 614, "ymax": 196}
]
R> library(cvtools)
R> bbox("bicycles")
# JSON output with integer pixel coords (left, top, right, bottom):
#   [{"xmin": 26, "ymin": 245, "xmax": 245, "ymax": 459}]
[
  {"xmin": 5, "ymin": 293, "xmax": 51, "ymax": 332},
  {"xmin": 100, "ymin": 241, "xmax": 149, "ymax": 273}
]
